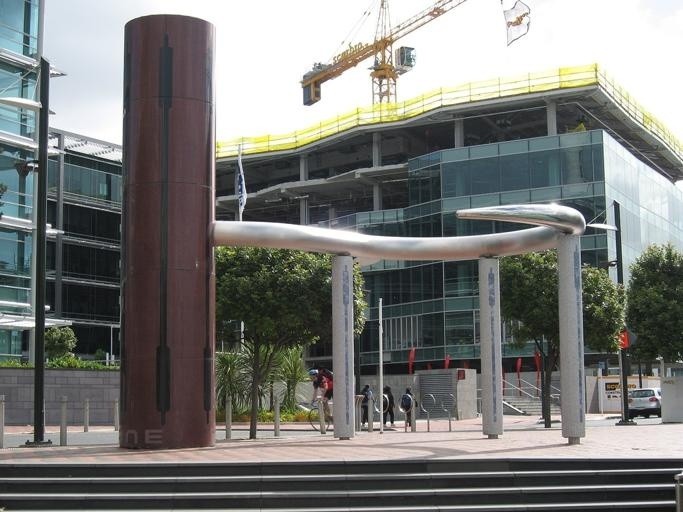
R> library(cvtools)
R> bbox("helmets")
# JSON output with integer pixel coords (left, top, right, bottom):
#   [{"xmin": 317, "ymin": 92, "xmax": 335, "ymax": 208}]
[{"xmin": 309, "ymin": 369, "xmax": 318, "ymax": 375}]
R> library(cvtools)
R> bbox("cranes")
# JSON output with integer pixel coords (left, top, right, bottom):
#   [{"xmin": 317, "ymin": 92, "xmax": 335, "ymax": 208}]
[{"xmin": 299, "ymin": 0, "xmax": 471, "ymax": 127}]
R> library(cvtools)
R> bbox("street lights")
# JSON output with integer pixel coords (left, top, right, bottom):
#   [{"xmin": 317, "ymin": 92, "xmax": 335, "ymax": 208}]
[
  {"xmin": 582, "ymin": 200, "xmax": 637, "ymax": 424},
  {"xmin": 0, "ymin": 54, "xmax": 54, "ymax": 447}
]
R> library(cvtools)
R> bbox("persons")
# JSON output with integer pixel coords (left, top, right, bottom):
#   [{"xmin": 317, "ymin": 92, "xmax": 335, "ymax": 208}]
[
  {"xmin": 383, "ymin": 385, "xmax": 396, "ymax": 427},
  {"xmin": 400, "ymin": 386, "xmax": 418, "ymax": 427},
  {"xmin": 360, "ymin": 385, "xmax": 373, "ymax": 426},
  {"xmin": 309, "ymin": 369, "xmax": 333, "ymax": 402}
]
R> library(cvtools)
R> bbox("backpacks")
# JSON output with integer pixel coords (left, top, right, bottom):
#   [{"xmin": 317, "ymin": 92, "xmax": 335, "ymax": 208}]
[
  {"xmin": 321, "ymin": 368, "xmax": 333, "ymax": 380},
  {"xmin": 402, "ymin": 395, "xmax": 411, "ymax": 406},
  {"xmin": 361, "ymin": 392, "xmax": 368, "ymax": 402}
]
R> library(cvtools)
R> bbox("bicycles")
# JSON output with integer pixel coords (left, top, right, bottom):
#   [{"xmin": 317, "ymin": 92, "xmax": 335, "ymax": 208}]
[{"xmin": 307, "ymin": 398, "xmax": 332, "ymax": 431}]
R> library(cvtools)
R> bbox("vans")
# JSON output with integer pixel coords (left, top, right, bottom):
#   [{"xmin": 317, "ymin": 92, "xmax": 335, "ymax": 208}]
[{"xmin": 626, "ymin": 387, "xmax": 661, "ymax": 420}]
